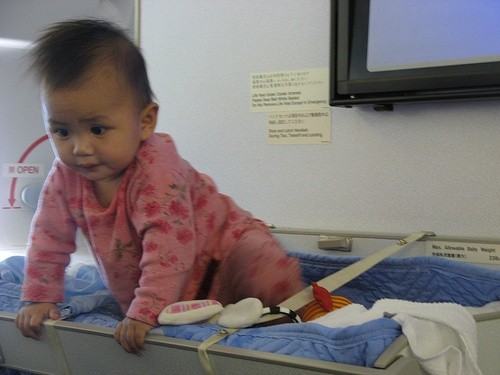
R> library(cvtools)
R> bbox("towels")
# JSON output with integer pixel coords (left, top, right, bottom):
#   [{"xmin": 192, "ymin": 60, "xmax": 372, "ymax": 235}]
[{"xmin": 302, "ymin": 299, "xmax": 485, "ymax": 375}]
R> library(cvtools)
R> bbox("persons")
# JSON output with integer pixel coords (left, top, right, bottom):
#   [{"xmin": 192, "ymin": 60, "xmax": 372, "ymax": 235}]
[{"xmin": 12, "ymin": 17, "xmax": 354, "ymax": 357}]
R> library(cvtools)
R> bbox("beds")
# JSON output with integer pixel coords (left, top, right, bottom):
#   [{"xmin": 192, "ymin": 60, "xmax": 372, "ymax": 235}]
[{"xmin": 0, "ymin": 226, "xmax": 500, "ymax": 374}]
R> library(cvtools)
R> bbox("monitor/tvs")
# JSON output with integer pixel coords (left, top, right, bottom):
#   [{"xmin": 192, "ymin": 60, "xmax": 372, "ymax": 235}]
[{"xmin": 328, "ymin": 1, "xmax": 500, "ymax": 104}]
